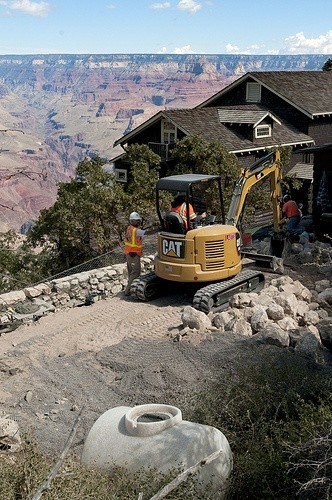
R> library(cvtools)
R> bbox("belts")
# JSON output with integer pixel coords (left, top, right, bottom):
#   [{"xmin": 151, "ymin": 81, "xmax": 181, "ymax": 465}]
[{"xmin": 290, "ymin": 214, "xmax": 299, "ymax": 218}]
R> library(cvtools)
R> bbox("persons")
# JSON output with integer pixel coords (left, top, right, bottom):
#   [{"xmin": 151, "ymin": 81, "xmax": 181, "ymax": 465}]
[
  {"xmin": 282, "ymin": 194, "xmax": 302, "ymax": 231},
  {"xmin": 170, "ymin": 187, "xmax": 206, "ymax": 232},
  {"xmin": 124, "ymin": 211, "xmax": 152, "ymax": 298}
]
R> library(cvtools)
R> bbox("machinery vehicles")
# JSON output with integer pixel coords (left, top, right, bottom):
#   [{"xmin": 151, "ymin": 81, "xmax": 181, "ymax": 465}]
[{"xmin": 130, "ymin": 151, "xmax": 292, "ymax": 315}]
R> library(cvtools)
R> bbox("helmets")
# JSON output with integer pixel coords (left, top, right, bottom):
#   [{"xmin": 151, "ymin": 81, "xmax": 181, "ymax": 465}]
[
  {"xmin": 128, "ymin": 212, "xmax": 142, "ymax": 220},
  {"xmin": 283, "ymin": 194, "xmax": 291, "ymax": 203},
  {"xmin": 178, "ymin": 191, "xmax": 192, "ymax": 197}
]
